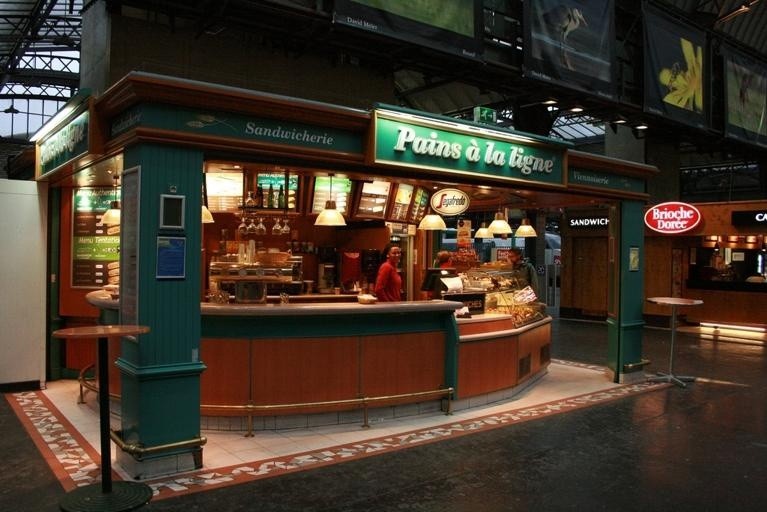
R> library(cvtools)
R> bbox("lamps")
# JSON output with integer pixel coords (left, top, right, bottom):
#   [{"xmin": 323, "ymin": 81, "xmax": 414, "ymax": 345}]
[
  {"xmin": 474, "ymin": 200, "xmax": 538, "ymax": 239},
  {"xmin": 99, "ymin": 175, "xmax": 120, "ymax": 225},
  {"xmin": 416, "ymin": 210, "xmax": 447, "ymax": 231},
  {"xmin": 312, "ymin": 172, "xmax": 349, "ymax": 228}
]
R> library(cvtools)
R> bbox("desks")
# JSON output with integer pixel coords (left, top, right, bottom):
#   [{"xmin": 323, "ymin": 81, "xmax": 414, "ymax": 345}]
[
  {"xmin": 49, "ymin": 324, "xmax": 153, "ymax": 512},
  {"xmin": 645, "ymin": 296, "xmax": 705, "ymax": 388}
]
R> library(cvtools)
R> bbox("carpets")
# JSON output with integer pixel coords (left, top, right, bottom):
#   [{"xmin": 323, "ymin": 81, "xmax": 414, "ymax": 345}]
[{"xmin": 4, "ymin": 354, "xmax": 678, "ymax": 503}]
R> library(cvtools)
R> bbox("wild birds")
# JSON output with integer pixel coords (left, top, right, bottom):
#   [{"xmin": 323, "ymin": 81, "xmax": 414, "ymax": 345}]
[{"xmin": 533, "ymin": 5, "xmax": 588, "ymax": 73}]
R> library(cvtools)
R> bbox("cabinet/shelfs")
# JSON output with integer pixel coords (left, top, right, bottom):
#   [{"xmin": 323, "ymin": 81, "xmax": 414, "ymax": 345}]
[{"xmin": 462, "ymin": 271, "xmax": 545, "ymax": 329}]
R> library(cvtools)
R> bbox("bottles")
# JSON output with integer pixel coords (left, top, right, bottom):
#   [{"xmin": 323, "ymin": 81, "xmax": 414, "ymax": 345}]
[{"xmin": 256, "ymin": 183, "xmax": 285, "ymax": 209}]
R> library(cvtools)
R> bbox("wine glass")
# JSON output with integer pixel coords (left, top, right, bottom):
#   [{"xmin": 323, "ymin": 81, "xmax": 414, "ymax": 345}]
[{"xmin": 238, "ymin": 217, "xmax": 290, "ymax": 235}]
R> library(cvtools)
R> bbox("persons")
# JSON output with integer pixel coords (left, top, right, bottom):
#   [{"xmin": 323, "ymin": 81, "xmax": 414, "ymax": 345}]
[
  {"xmin": 508, "ymin": 248, "xmax": 538, "ymax": 300},
  {"xmin": 375, "ymin": 243, "xmax": 401, "ymax": 301},
  {"xmin": 427, "ymin": 251, "xmax": 458, "ymax": 299}
]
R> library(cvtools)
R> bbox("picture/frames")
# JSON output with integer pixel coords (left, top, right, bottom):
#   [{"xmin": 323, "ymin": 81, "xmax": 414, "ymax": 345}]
[
  {"xmin": 628, "ymin": 245, "xmax": 641, "ymax": 272},
  {"xmin": 118, "ymin": 164, "xmax": 142, "ymax": 344},
  {"xmin": 154, "ymin": 192, "xmax": 187, "ymax": 281}
]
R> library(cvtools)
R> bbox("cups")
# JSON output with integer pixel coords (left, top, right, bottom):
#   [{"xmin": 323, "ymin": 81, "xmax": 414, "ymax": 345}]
[{"xmin": 236, "ymin": 239, "xmax": 257, "ymax": 263}]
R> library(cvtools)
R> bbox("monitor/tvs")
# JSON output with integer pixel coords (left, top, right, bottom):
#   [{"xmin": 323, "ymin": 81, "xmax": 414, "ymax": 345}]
[{"xmin": 421, "ymin": 268, "xmax": 458, "ymax": 301}]
